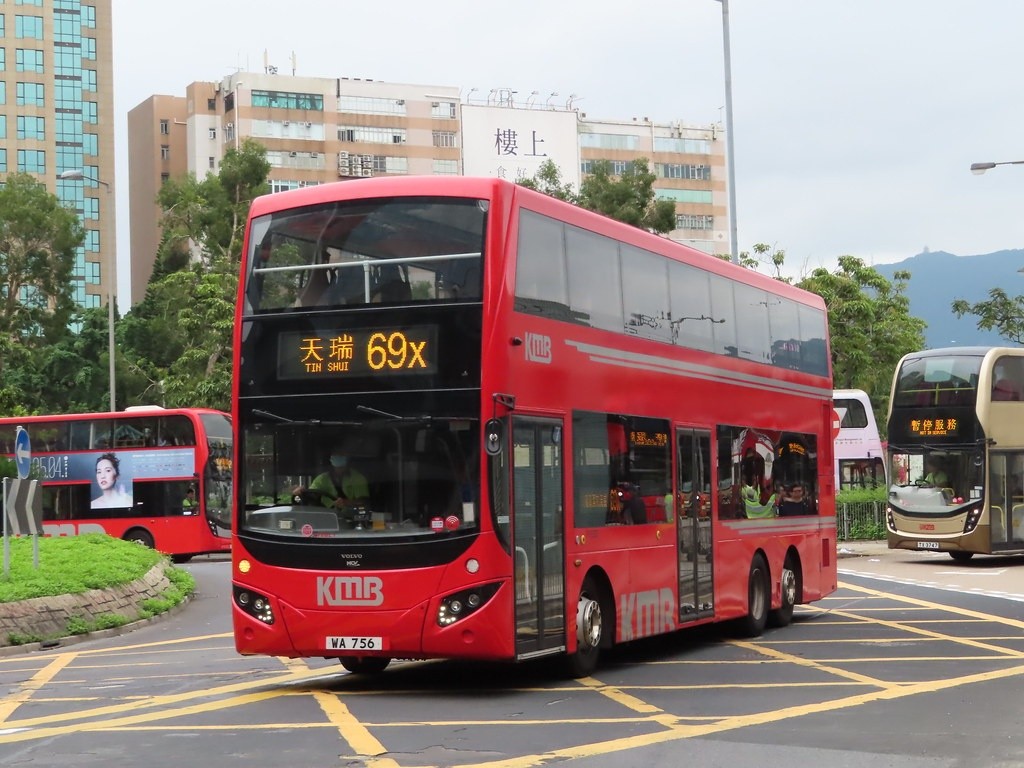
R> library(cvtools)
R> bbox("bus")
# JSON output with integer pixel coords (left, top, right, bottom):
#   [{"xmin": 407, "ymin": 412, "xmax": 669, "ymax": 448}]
[
  {"xmin": 883, "ymin": 345, "xmax": 1024, "ymax": 567},
  {"xmin": 0, "ymin": 405, "xmax": 233, "ymax": 566},
  {"xmin": 226, "ymin": 174, "xmax": 839, "ymax": 679},
  {"xmin": 830, "ymin": 389, "xmax": 888, "ymax": 498}
]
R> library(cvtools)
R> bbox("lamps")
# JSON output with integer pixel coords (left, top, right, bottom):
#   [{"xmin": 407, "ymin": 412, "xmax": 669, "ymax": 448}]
[
  {"xmin": 506, "ymin": 91, "xmax": 518, "ymax": 106},
  {"xmin": 545, "ymin": 92, "xmax": 559, "ymax": 106},
  {"xmin": 565, "ymin": 93, "xmax": 577, "ymax": 109},
  {"xmin": 467, "ymin": 87, "xmax": 477, "ymax": 105},
  {"xmin": 487, "ymin": 88, "xmax": 496, "ymax": 106},
  {"xmin": 524, "ymin": 90, "xmax": 538, "ymax": 108}
]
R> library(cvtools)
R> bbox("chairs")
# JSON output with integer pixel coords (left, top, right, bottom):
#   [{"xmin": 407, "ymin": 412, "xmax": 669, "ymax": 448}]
[
  {"xmin": 375, "ymin": 257, "xmax": 411, "ymax": 301},
  {"xmin": 331, "ymin": 258, "xmax": 384, "ymax": 304}
]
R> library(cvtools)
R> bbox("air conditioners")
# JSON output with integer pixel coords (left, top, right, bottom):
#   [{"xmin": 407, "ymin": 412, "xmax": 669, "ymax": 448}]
[
  {"xmin": 228, "ymin": 123, "xmax": 232, "ymax": 128},
  {"xmin": 432, "ymin": 101, "xmax": 439, "ymax": 107},
  {"xmin": 311, "ymin": 151, "xmax": 319, "ymax": 158},
  {"xmin": 222, "ymin": 125, "xmax": 227, "ymax": 129},
  {"xmin": 708, "ymin": 216, "xmax": 713, "ymax": 222},
  {"xmin": 351, "ymin": 157, "xmax": 361, "ymax": 164},
  {"xmin": 339, "ymin": 159, "xmax": 350, "ymax": 167},
  {"xmin": 398, "ymin": 99, "xmax": 404, "ymax": 105},
  {"xmin": 363, "ymin": 155, "xmax": 372, "ymax": 162},
  {"xmin": 283, "ymin": 120, "xmax": 290, "ymax": 125},
  {"xmin": 363, "ymin": 169, "xmax": 371, "ymax": 176},
  {"xmin": 678, "ymin": 216, "xmax": 685, "ymax": 221},
  {"xmin": 352, "ymin": 171, "xmax": 362, "ymax": 176},
  {"xmin": 696, "ymin": 163, "xmax": 704, "ymax": 170},
  {"xmin": 352, "ymin": 165, "xmax": 362, "ymax": 171},
  {"xmin": 339, "ymin": 151, "xmax": 350, "ymax": 159},
  {"xmin": 362, "ymin": 162, "xmax": 372, "ymax": 169},
  {"xmin": 306, "ymin": 121, "xmax": 311, "ymax": 127},
  {"xmin": 290, "ymin": 150, "xmax": 296, "ymax": 157},
  {"xmin": 338, "ymin": 167, "xmax": 349, "ymax": 177}
]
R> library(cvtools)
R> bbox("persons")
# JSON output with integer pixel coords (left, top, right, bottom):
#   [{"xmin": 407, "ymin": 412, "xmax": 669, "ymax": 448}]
[
  {"xmin": 91, "ymin": 454, "xmax": 133, "ymax": 509},
  {"xmin": 617, "ymin": 482, "xmax": 647, "ymax": 523},
  {"xmin": 922, "ymin": 463, "xmax": 948, "ymax": 488},
  {"xmin": 740, "ymin": 476, "xmax": 810, "ymax": 518},
  {"xmin": 293, "ymin": 449, "xmax": 369, "ymax": 511},
  {"xmin": 183, "ymin": 489, "xmax": 199, "ymax": 516},
  {"xmin": 451, "ymin": 462, "xmax": 490, "ymax": 507}
]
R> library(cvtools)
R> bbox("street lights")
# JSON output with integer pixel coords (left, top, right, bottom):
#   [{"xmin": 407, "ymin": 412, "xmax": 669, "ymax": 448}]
[{"xmin": 56, "ymin": 170, "xmax": 118, "ymax": 448}]
